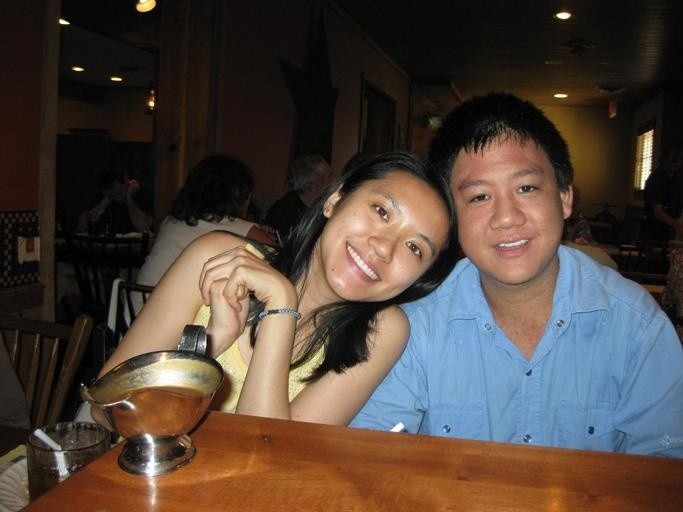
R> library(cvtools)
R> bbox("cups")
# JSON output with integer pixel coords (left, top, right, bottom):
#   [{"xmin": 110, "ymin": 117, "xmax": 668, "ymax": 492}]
[
  {"xmin": 25, "ymin": 421, "xmax": 112, "ymax": 504},
  {"xmin": 79, "ymin": 322, "xmax": 226, "ymax": 477}
]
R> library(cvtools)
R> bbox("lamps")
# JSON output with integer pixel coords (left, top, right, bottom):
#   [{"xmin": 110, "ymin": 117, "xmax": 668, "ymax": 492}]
[{"xmin": 143, "ymin": 80, "xmax": 160, "ymax": 115}]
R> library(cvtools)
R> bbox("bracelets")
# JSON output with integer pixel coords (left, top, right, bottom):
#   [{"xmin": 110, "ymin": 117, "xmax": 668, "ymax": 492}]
[{"xmin": 259, "ymin": 307, "xmax": 305, "ymax": 316}]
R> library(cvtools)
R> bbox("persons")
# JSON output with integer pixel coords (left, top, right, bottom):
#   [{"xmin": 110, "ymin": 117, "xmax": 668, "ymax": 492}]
[
  {"xmin": 90, "ymin": 149, "xmax": 456, "ymax": 438},
  {"xmin": 0, "ymin": 328, "xmax": 33, "ymax": 463},
  {"xmin": 80, "ymin": 151, "xmax": 337, "ymax": 339},
  {"xmin": 346, "ymin": 91, "xmax": 683, "ymax": 460},
  {"xmin": 646, "ymin": 151, "xmax": 682, "ymax": 246}
]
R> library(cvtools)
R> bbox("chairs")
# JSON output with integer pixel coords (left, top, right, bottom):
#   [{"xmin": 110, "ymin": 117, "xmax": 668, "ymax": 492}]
[
  {"xmin": 64, "ymin": 231, "xmax": 150, "ymax": 324},
  {"xmin": 615, "ymin": 247, "xmax": 644, "ymax": 272},
  {"xmin": 111, "ymin": 281, "xmax": 156, "ymax": 348},
  {"xmin": 0, "ymin": 308, "xmax": 94, "ymax": 429}
]
